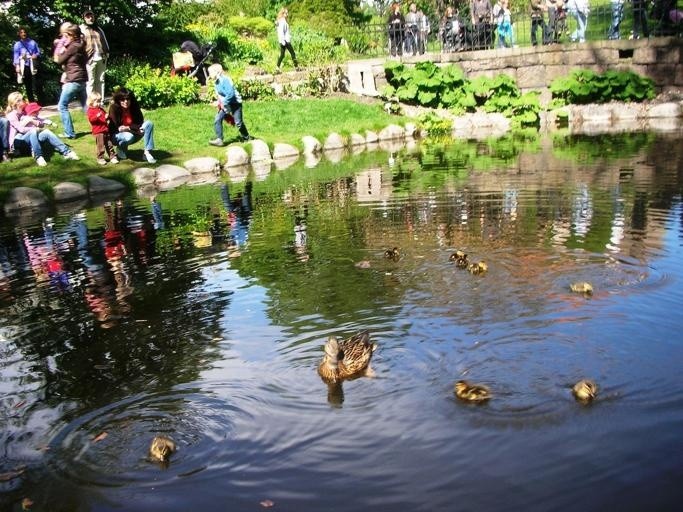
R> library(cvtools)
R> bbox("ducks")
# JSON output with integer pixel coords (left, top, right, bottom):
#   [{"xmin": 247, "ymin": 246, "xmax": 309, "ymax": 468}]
[
  {"xmin": 468, "ymin": 260, "xmax": 488, "ymax": 276},
  {"xmin": 572, "ymin": 380, "xmax": 598, "ymax": 402},
  {"xmin": 455, "ymin": 253, "xmax": 470, "ymax": 269},
  {"xmin": 317, "ymin": 329, "xmax": 376, "ymax": 378},
  {"xmin": 384, "ymin": 245, "xmax": 400, "ymax": 259},
  {"xmin": 448, "ymin": 250, "xmax": 464, "ymax": 263},
  {"xmin": 150, "ymin": 434, "xmax": 175, "ymax": 462},
  {"xmin": 569, "ymin": 280, "xmax": 593, "ymax": 293},
  {"xmin": 454, "ymin": 380, "xmax": 494, "ymax": 401}
]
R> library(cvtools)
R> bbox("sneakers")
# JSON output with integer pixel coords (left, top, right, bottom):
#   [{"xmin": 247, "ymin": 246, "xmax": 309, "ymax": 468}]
[
  {"xmin": 58, "ymin": 132, "xmax": 77, "ymax": 139},
  {"xmin": 2, "ymin": 152, "xmax": 12, "ymax": 163},
  {"xmin": 35, "ymin": 155, "xmax": 47, "ymax": 167},
  {"xmin": 110, "ymin": 156, "xmax": 119, "ymax": 164},
  {"xmin": 63, "ymin": 150, "xmax": 80, "ymax": 161},
  {"xmin": 115, "ymin": 146, "xmax": 128, "ymax": 159},
  {"xmin": 142, "ymin": 152, "xmax": 157, "ymax": 164},
  {"xmin": 208, "ymin": 137, "xmax": 224, "ymax": 147},
  {"xmin": 97, "ymin": 159, "xmax": 107, "ymax": 165},
  {"xmin": 243, "ymin": 134, "xmax": 251, "ymax": 144}
]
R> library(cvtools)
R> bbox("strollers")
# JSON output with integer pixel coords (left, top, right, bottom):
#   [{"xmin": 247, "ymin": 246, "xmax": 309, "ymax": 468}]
[
  {"xmin": 171, "ymin": 39, "xmax": 216, "ymax": 86},
  {"xmin": 390, "ymin": 24, "xmax": 427, "ymax": 56}
]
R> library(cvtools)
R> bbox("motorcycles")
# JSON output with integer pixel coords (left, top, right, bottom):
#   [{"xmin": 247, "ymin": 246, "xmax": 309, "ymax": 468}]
[
  {"xmin": 649, "ymin": 2, "xmax": 682, "ymax": 38},
  {"xmin": 440, "ymin": 15, "xmax": 498, "ymax": 54},
  {"xmin": 548, "ymin": 0, "xmax": 570, "ymax": 46}
]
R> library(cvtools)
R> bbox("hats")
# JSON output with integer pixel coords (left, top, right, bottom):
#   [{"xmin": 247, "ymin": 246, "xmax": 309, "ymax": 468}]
[
  {"xmin": 58, "ymin": 21, "xmax": 74, "ymax": 37},
  {"xmin": 25, "ymin": 102, "xmax": 42, "ymax": 116},
  {"xmin": 82, "ymin": 9, "xmax": 95, "ymax": 19}
]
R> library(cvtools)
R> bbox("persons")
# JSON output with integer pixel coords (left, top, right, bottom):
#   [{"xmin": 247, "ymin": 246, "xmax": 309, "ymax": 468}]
[
  {"xmin": 275, "ymin": 8, "xmax": 303, "ymax": 73},
  {"xmin": 76, "ymin": 10, "xmax": 110, "ymax": 105},
  {"xmin": 1, "ymin": 87, "xmax": 160, "ymax": 170},
  {"xmin": 384, "ymin": 0, "xmax": 683, "ymax": 56},
  {"xmin": 55, "ymin": 26, "xmax": 96, "ymax": 139},
  {"xmin": 208, "ymin": 63, "xmax": 250, "ymax": 147},
  {"xmin": 52, "ymin": 20, "xmax": 75, "ymax": 83},
  {"xmin": 13, "ymin": 28, "xmax": 46, "ymax": 107}
]
